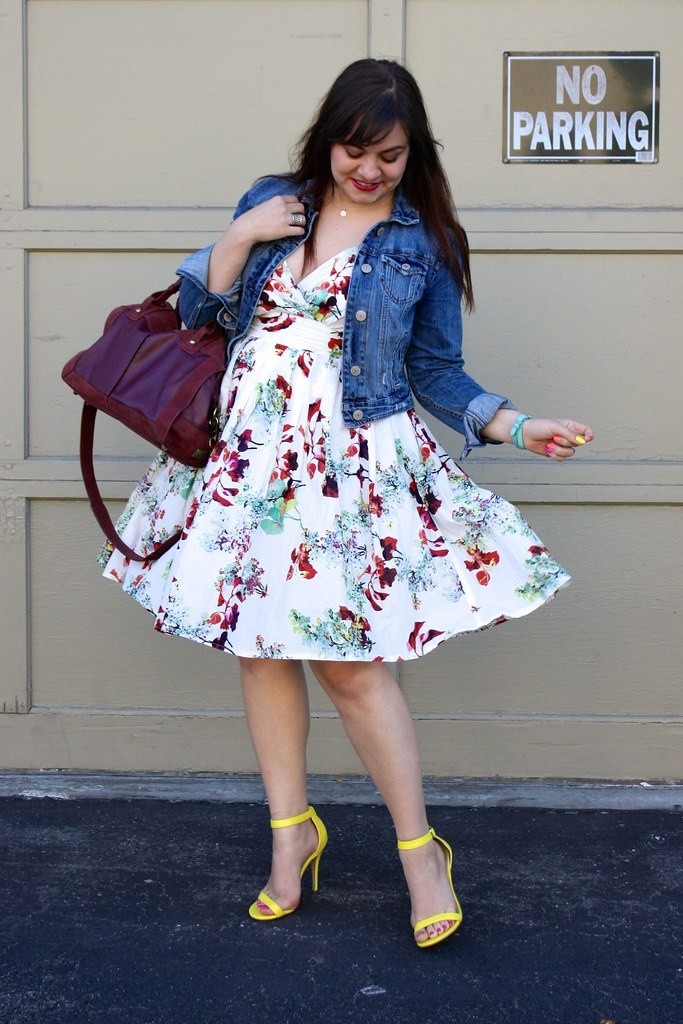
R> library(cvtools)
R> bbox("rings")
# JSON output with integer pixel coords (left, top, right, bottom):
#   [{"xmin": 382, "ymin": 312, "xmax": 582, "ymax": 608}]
[{"xmin": 292, "ymin": 214, "xmax": 303, "ymax": 225}]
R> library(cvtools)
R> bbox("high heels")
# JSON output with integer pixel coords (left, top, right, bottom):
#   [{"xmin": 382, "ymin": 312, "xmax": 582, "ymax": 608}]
[
  {"xmin": 248, "ymin": 805, "xmax": 329, "ymax": 921},
  {"xmin": 397, "ymin": 825, "xmax": 463, "ymax": 949}
]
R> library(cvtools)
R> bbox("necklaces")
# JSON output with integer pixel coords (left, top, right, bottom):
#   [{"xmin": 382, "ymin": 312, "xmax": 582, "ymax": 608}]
[{"xmin": 339, "ymin": 206, "xmax": 349, "ymax": 217}]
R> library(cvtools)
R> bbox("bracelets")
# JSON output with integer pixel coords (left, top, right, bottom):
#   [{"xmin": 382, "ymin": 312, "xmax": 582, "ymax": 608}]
[{"xmin": 511, "ymin": 414, "xmax": 532, "ymax": 449}]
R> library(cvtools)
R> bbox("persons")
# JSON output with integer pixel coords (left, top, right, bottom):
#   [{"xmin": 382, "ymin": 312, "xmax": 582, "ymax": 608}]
[{"xmin": 97, "ymin": 59, "xmax": 593, "ymax": 946}]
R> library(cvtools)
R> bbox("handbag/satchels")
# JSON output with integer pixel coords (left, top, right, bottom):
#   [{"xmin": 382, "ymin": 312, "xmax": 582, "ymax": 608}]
[{"xmin": 59, "ymin": 273, "xmax": 231, "ymax": 564}]
